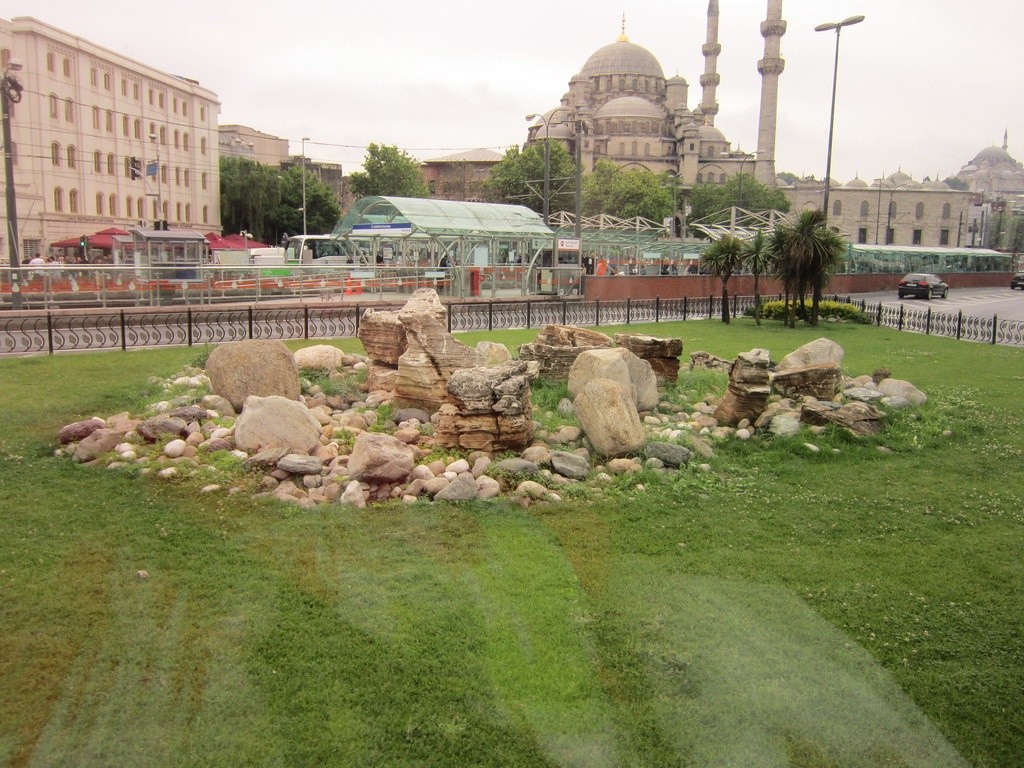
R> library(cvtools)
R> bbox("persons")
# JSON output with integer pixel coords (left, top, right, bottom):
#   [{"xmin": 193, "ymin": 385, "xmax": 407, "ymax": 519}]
[
  {"xmin": 21, "ymin": 252, "xmax": 114, "ymax": 289},
  {"xmin": 322, "ymin": 249, "xmax": 700, "ymax": 280}
]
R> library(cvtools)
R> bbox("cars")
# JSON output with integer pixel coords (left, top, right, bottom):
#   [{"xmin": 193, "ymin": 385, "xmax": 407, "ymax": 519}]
[
  {"xmin": 899, "ymin": 273, "xmax": 949, "ymax": 300},
  {"xmin": 1009, "ymin": 272, "xmax": 1024, "ymax": 289}
]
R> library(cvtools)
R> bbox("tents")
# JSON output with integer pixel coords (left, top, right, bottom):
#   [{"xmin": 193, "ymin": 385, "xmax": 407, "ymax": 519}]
[{"xmin": 49, "ymin": 227, "xmax": 264, "ymax": 251}]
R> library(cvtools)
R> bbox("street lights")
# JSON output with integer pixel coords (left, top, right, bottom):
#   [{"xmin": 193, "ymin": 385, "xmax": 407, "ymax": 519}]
[
  {"xmin": 566, "ymin": 104, "xmax": 600, "ymax": 236},
  {"xmin": 809, "ymin": 15, "xmax": 867, "ymax": 323},
  {"xmin": 886, "ymin": 183, "xmax": 914, "ymax": 245},
  {"xmin": 0, "ymin": 58, "xmax": 24, "ymax": 310},
  {"xmin": 737, "ymin": 150, "xmax": 766, "ymax": 230},
  {"xmin": 525, "ymin": 106, "xmax": 574, "ymax": 227},
  {"xmin": 149, "ymin": 133, "xmax": 164, "ymax": 232},
  {"xmin": 298, "ymin": 137, "xmax": 311, "ymax": 235},
  {"xmin": 239, "ymin": 230, "xmax": 253, "ymax": 261},
  {"xmin": 667, "ymin": 171, "xmax": 683, "ymax": 275},
  {"xmin": 873, "ymin": 178, "xmax": 882, "ymax": 245}
]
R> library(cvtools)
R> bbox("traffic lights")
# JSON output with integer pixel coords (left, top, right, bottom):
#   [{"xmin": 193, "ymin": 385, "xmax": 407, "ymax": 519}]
[{"xmin": 80, "ymin": 236, "xmax": 86, "ymax": 247}]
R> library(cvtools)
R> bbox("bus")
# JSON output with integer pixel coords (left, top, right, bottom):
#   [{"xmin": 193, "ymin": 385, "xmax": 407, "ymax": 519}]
[{"xmin": 284, "ymin": 233, "xmax": 458, "ymax": 268}]
